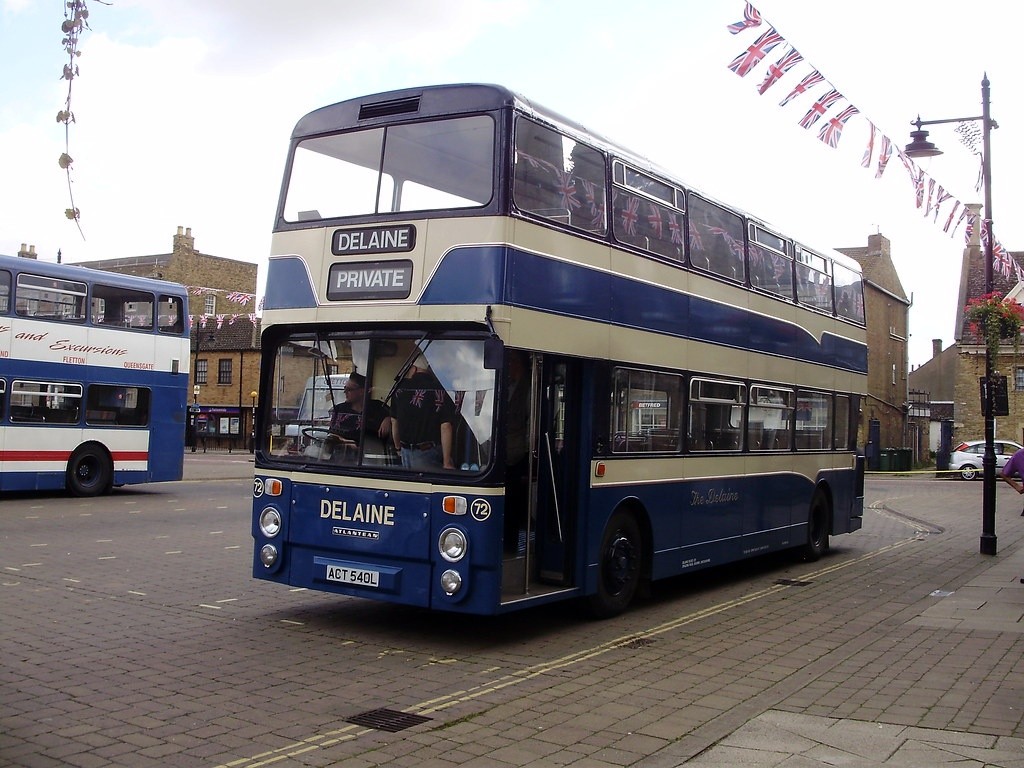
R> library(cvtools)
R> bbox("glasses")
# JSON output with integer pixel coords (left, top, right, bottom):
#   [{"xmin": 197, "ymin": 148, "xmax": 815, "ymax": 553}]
[{"xmin": 344, "ymin": 385, "xmax": 360, "ymax": 391}]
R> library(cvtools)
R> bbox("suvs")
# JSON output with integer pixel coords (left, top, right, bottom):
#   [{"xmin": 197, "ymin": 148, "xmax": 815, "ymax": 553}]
[{"xmin": 948, "ymin": 440, "xmax": 1024, "ymax": 481}]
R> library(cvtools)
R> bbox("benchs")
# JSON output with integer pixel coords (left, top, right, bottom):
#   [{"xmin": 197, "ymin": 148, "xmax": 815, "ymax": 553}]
[
  {"xmin": 0, "ymin": 305, "xmax": 132, "ymax": 328},
  {"xmin": 612, "ymin": 428, "xmax": 821, "ymax": 453},
  {"xmin": 510, "ymin": 151, "xmax": 818, "ymax": 307},
  {"xmin": 8, "ymin": 404, "xmax": 116, "ymax": 424}
]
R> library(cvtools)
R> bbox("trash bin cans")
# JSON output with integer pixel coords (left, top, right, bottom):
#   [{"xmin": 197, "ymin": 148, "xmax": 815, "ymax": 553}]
[{"xmin": 880, "ymin": 447, "xmax": 913, "ymax": 471}]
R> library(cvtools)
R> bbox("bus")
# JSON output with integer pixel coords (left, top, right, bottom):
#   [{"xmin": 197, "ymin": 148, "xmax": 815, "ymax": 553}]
[
  {"xmin": 0, "ymin": 255, "xmax": 191, "ymax": 496},
  {"xmin": 297, "ymin": 374, "xmax": 350, "ymax": 420},
  {"xmin": 250, "ymin": 81, "xmax": 868, "ymax": 619}
]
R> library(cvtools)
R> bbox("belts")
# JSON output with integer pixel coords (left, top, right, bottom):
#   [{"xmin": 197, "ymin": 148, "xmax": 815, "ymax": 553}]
[{"xmin": 400, "ymin": 442, "xmax": 441, "ymax": 450}]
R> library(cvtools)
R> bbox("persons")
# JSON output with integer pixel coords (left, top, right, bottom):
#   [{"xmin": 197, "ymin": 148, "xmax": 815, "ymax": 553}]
[
  {"xmin": 1002, "ymin": 450, "xmax": 1024, "ymax": 583},
  {"xmin": 390, "ymin": 356, "xmax": 457, "ymax": 469},
  {"xmin": 327, "ymin": 372, "xmax": 391, "ymax": 443}
]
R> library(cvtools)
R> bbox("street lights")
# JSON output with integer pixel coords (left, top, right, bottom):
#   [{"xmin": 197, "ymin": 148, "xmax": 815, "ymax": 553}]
[
  {"xmin": 898, "ymin": 71, "xmax": 998, "ymax": 556},
  {"xmin": 250, "ymin": 391, "xmax": 257, "ymax": 436},
  {"xmin": 191, "ymin": 321, "xmax": 216, "ymax": 451}
]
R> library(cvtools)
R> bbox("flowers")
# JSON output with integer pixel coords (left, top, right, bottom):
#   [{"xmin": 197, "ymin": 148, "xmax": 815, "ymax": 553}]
[{"xmin": 963, "ymin": 291, "xmax": 1024, "ymax": 414}]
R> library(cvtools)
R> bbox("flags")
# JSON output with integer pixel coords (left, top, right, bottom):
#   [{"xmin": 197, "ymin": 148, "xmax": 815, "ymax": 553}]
[
  {"xmin": 779, "ymin": 70, "xmax": 825, "ymax": 107},
  {"xmin": 756, "ymin": 47, "xmax": 804, "ymax": 95},
  {"xmin": 517, "ymin": 149, "xmax": 864, "ymax": 316},
  {"xmin": 798, "ymin": 88, "xmax": 843, "ymax": 130},
  {"xmin": 185, "ymin": 285, "xmax": 253, "ymax": 306},
  {"xmin": 860, "ymin": 122, "xmax": 989, "ymax": 246},
  {"xmin": 729, "ymin": 27, "xmax": 785, "ymax": 78},
  {"xmin": 993, "ymin": 235, "xmax": 1024, "ymax": 281},
  {"xmin": 782, "ymin": 402, "xmax": 812, "ymax": 420},
  {"xmin": 330, "ymin": 413, "xmax": 363, "ymax": 430},
  {"xmin": 125, "ymin": 314, "xmax": 258, "ymax": 327},
  {"xmin": 727, "ymin": 1, "xmax": 762, "ymax": 35},
  {"xmin": 817, "ymin": 105, "xmax": 860, "ymax": 149},
  {"xmin": 395, "ymin": 389, "xmax": 487, "ymax": 416}
]
